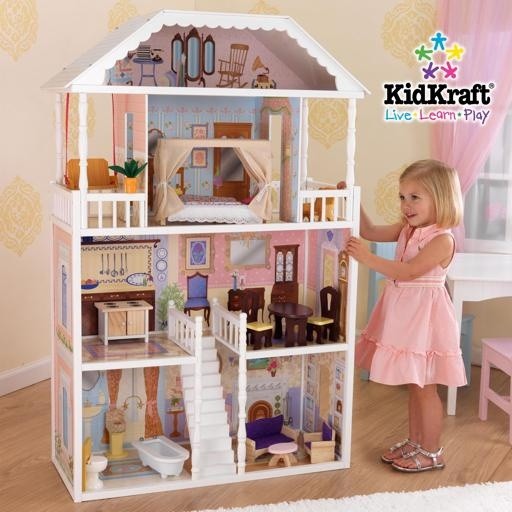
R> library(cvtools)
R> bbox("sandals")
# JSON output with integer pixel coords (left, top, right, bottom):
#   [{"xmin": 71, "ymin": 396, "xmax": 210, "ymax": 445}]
[
  {"xmin": 392, "ymin": 444, "xmax": 448, "ymax": 473},
  {"xmin": 380, "ymin": 437, "xmax": 424, "ymax": 464}
]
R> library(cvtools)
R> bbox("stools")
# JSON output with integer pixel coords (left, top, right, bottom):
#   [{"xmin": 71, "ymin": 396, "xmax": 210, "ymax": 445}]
[{"xmin": 478, "ymin": 338, "xmax": 512, "ymax": 445}]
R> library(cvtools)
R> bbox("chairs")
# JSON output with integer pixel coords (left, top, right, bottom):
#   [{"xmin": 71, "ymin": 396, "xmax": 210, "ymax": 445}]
[{"xmin": 360, "ymin": 241, "xmax": 474, "ymax": 385}]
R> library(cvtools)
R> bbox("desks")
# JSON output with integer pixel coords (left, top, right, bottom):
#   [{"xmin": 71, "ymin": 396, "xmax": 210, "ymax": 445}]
[{"xmin": 447, "ymin": 252, "xmax": 512, "ymax": 415}]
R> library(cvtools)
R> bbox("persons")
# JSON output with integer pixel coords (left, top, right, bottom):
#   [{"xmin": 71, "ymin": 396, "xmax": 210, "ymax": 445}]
[
  {"xmin": 336, "ymin": 159, "xmax": 465, "ymax": 471},
  {"xmin": 59, "ymin": 28, "xmax": 348, "ymax": 493}
]
